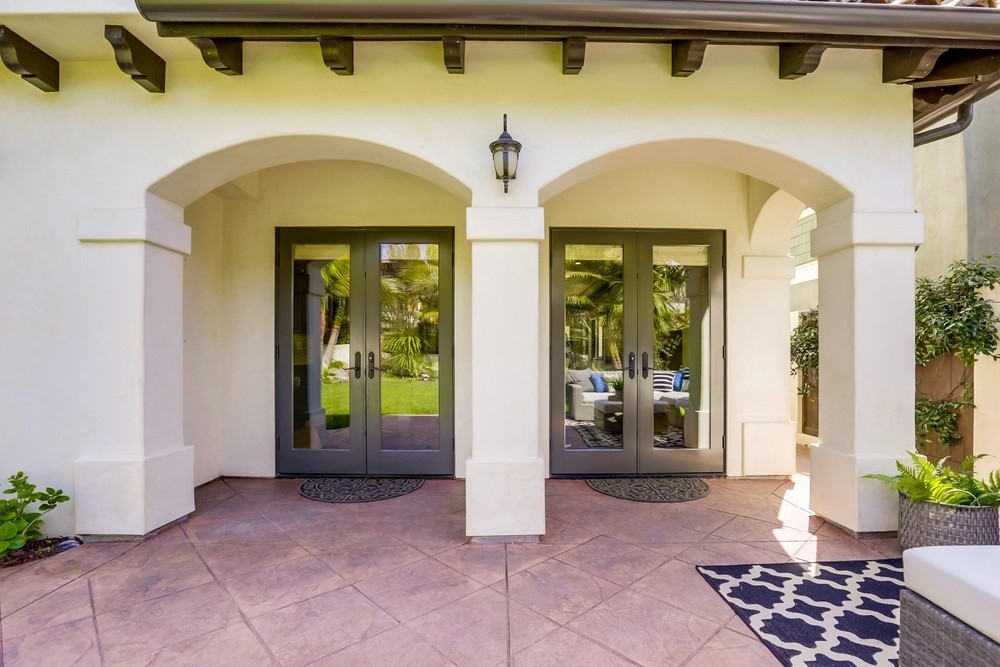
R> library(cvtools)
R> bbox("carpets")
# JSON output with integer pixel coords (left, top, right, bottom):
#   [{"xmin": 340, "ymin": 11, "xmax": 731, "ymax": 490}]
[
  {"xmin": 299, "ymin": 476, "xmax": 424, "ymax": 503},
  {"xmin": 566, "ymin": 410, "xmax": 687, "ymax": 448},
  {"xmin": 583, "ymin": 478, "xmax": 709, "ymax": 502},
  {"xmin": 696, "ymin": 559, "xmax": 908, "ymax": 667}
]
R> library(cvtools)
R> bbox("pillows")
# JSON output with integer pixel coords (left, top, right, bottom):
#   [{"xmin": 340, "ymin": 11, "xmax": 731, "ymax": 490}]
[
  {"xmin": 590, "ymin": 372, "xmax": 607, "ymax": 392},
  {"xmin": 652, "ymin": 372, "xmax": 676, "ymax": 391},
  {"xmin": 603, "ymin": 372, "xmax": 624, "ymax": 393},
  {"xmin": 567, "ymin": 367, "xmax": 594, "ymax": 392},
  {"xmin": 673, "ymin": 367, "xmax": 691, "ymax": 391}
]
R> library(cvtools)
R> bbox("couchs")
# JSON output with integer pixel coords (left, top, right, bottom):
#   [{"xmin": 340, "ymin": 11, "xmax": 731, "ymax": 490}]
[{"xmin": 567, "ymin": 372, "xmax": 691, "ymax": 421}]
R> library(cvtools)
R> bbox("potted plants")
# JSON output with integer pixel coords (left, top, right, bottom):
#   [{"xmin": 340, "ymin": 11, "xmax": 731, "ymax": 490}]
[
  {"xmin": 609, "ymin": 377, "xmax": 625, "ymax": 398},
  {"xmin": 857, "ymin": 450, "xmax": 1000, "ymax": 553}
]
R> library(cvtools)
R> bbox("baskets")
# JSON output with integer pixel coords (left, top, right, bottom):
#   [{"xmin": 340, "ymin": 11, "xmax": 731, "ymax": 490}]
[{"xmin": 897, "ymin": 488, "xmax": 1000, "ymax": 551}]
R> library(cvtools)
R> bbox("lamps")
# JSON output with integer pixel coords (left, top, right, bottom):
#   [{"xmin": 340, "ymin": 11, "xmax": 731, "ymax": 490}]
[{"xmin": 488, "ymin": 113, "xmax": 522, "ymax": 194}]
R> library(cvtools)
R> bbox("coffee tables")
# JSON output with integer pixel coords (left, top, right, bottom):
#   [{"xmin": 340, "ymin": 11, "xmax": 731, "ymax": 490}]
[{"xmin": 594, "ymin": 400, "xmax": 669, "ymax": 432}]
